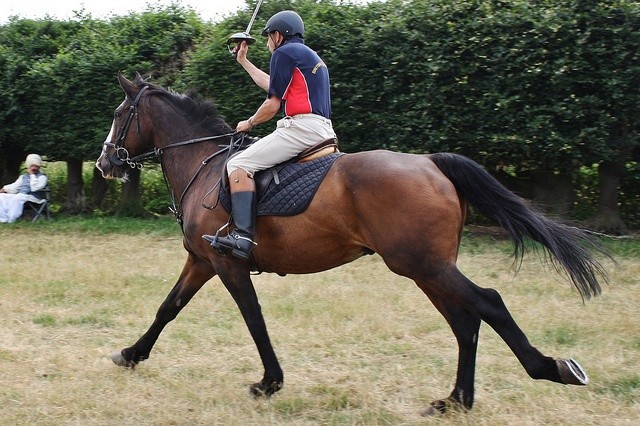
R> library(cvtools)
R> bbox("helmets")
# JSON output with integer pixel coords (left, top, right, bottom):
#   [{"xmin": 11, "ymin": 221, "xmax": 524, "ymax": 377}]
[{"xmin": 261, "ymin": 11, "xmax": 304, "ymax": 36}]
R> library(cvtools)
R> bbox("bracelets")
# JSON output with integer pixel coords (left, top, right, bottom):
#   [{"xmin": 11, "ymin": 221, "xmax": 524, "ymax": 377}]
[{"xmin": 247, "ymin": 118, "xmax": 254, "ymax": 128}]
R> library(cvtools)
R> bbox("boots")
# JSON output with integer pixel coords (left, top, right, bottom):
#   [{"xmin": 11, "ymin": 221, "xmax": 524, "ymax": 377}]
[{"xmin": 202, "ymin": 191, "xmax": 255, "ymax": 262}]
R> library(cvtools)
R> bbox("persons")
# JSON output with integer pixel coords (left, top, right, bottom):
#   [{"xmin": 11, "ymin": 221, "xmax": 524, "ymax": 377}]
[
  {"xmin": 201, "ymin": 10, "xmax": 340, "ymax": 263},
  {"xmin": 0, "ymin": 153, "xmax": 49, "ymax": 222}
]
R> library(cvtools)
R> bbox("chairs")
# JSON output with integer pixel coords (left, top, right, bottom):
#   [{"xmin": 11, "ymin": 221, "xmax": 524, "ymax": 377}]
[{"xmin": 24, "ymin": 176, "xmax": 51, "ymax": 226}]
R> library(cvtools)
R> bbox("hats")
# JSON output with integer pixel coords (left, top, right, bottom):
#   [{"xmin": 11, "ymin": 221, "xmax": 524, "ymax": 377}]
[{"xmin": 25, "ymin": 153, "xmax": 41, "ymax": 166}]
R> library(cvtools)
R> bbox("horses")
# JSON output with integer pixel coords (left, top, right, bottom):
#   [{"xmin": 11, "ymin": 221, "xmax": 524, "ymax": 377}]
[{"xmin": 94, "ymin": 70, "xmax": 624, "ymax": 418}]
